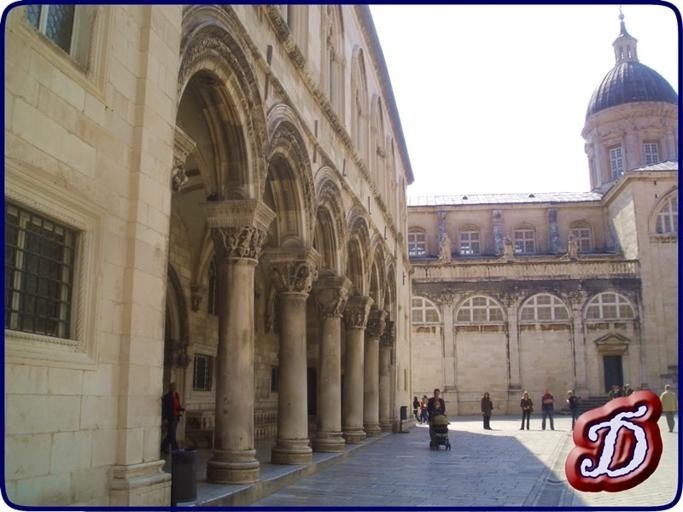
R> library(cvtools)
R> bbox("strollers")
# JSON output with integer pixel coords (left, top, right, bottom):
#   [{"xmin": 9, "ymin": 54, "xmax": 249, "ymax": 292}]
[{"xmin": 426, "ymin": 405, "xmax": 452, "ymax": 451}]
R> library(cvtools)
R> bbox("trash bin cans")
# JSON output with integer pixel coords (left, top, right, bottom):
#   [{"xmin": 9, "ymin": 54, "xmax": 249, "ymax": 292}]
[{"xmin": 171, "ymin": 448, "xmax": 197, "ymax": 506}]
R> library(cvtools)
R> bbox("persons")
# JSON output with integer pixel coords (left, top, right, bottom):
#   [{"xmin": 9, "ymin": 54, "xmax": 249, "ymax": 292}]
[
  {"xmin": 612, "ymin": 383, "xmax": 625, "ymax": 400},
  {"xmin": 518, "ymin": 392, "xmax": 533, "ymax": 430},
  {"xmin": 607, "ymin": 385, "xmax": 616, "ymax": 401},
  {"xmin": 412, "ymin": 396, "xmax": 421, "ymax": 424},
  {"xmin": 430, "ymin": 400, "xmax": 443, "ymax": 420},
  {"xmin": 657, "ymin": 384, "xmax": 677, "ymax": 432},
  {"xmin": 565, "ymin": 389, "xmax": 580, "ymax": 430},
  {"xmin": 425, "ymin": 388, "xmax": 445, "ymax": 427},
  {"xmin": 422, "ymin": 395, "xmax": 429, "ymax": 417},
  {"xmin": 158, "ymin": 380, "xmax": 186, "ymax": 452},
  {"xmin": 479, "ymin": 391, "xmax": 494, "ymax": 430},
  {"xmin": 622, "ymin": 383, "xmax": 633, "ymax": 398},
  {"xmin": 418, "ymin": 398, "xmax": 429, "ymax": 424},
  {"xmin": 540, "ymin": 387, "xmax": 555, "ymax": 431}
]
